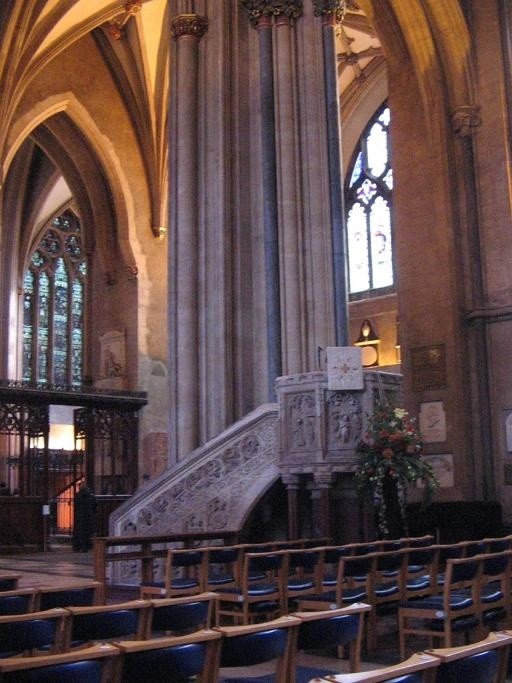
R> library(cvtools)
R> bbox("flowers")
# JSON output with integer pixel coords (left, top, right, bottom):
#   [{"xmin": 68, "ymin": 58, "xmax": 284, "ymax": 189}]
[{"xmin": 352, "ymin": 398, "xmax": 440, "ymax": 535}]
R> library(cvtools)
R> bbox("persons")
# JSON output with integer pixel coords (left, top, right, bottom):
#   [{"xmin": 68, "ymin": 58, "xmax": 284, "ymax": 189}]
[
  {"xmin": 138, "ymin": 472, "xmax": 151, "ymax": 489},
  {"xmin": 24, "ymin": 480, "xmax": 33, "ymax": 496},
  {"xmin": 73, "ymin": 485, "xmax": 96, "ymax": 552},
  {"xmin": 102, "ymin": 480, "xmax": 116, "ymax": 495},
  {"xmin": 13, "ymin": 480, "xmax": 19, "ymax": 496}
]
